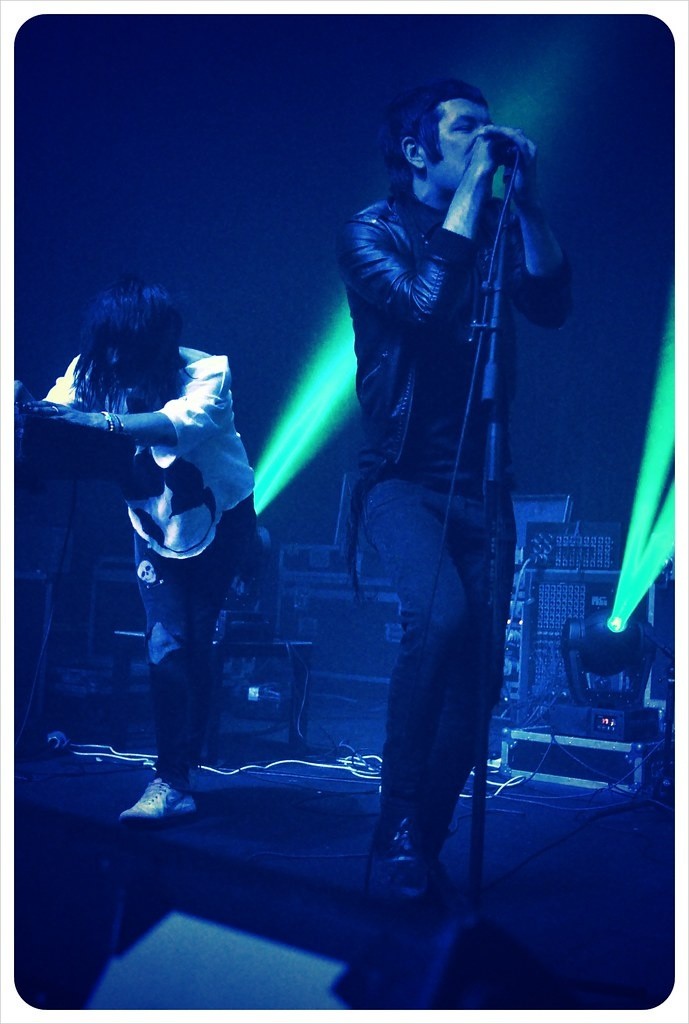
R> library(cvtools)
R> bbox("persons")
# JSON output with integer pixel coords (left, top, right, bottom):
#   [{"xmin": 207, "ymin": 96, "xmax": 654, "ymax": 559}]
[
  {"xmin": 336, "ymin": 69, "xmax": 577, "ymax": 919},
  {"xmin": 13, "ymin": 277, "xmax": 291, "ymax": 844}
]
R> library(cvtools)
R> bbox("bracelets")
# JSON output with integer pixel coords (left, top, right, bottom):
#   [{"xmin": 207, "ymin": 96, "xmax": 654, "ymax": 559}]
[
  {"xmin": 101, "ymin": 411, "xmax": 116, "ymax": 436},
  {"xmin": 114, "ymin": 415, "xmax": 125, "ymax": 436}
]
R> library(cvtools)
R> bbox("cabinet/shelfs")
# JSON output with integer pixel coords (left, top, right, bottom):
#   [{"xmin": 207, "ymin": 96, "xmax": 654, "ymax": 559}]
[
  {"xmin": 502, "ymin": 720, "xmax": 667, "ymax": 795},
  {"xmin": 273, "ymin": 469, "xmax": 573, "ymax": 684}
]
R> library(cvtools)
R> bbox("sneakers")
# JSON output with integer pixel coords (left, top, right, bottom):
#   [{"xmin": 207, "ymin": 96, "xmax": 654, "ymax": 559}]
[{"xmin": 120, "ymin": 779, "xmax": 198, "ymax": 828}]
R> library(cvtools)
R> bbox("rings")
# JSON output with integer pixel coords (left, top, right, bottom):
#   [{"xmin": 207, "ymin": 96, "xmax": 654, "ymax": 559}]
[{"xmin": 50, "ymin": 405, "xmax": 60, "ymax": 416}]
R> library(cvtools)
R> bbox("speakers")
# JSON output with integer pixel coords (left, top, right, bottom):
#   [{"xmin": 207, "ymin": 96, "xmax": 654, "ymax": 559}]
[{"xmin": 524, "ymin": 521, "xmax": 655, "ymax": 738}]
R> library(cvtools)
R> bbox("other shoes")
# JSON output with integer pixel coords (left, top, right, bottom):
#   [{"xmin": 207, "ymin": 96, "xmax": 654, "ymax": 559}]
[{"xmin": 367, "ymin": 828, "xmax": 429, "ymax": 887}]
